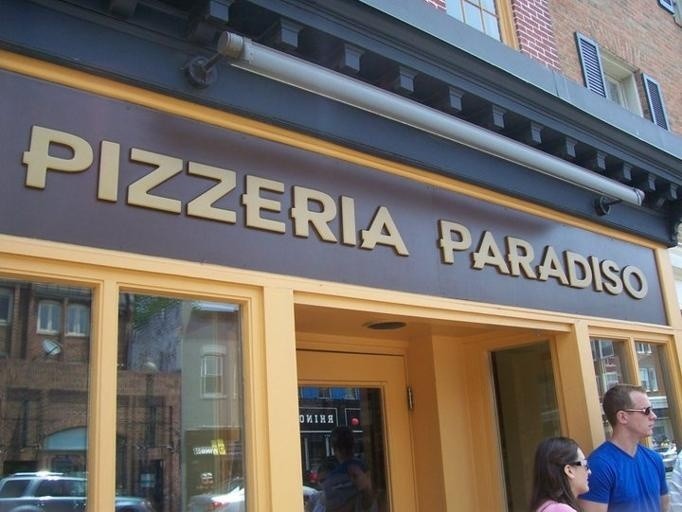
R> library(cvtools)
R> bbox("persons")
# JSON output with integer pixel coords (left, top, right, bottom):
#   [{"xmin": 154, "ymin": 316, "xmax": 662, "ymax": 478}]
[
  {"xmin": 302, "ymin": 426, "xmax": 387, "ymax": 510},
  {"xmin": 652, "ymin": 435, "xmax": 677, "ymax": 461},
  {"xmin": 666, "ymin": 451, "xmax": 682, "ymax": 512},
  {"xmin": 577, "ymin": 383, "xmax": 673, "ymax": 511},
  {"xmin": 530, "ymin": 436, "xmax": 591, "ymax": 512}
]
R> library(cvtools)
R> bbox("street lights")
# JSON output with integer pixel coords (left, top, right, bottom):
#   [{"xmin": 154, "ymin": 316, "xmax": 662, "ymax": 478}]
[{"xmin": 141, "ymin": 359, "xmax": 159, "ymax": 447}]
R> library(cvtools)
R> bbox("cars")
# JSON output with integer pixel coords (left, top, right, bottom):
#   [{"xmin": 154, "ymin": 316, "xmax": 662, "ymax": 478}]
[
  {"xmin": 304, "ymin": 456, "xmax": 368, "ymax": 488},
  {"xmin": 655, "ymin": 445, "xmax": 678, "ymax": 471},
  {"xmin": 188, "ymin": 477, "xmax": 320, "ymax": 511}
]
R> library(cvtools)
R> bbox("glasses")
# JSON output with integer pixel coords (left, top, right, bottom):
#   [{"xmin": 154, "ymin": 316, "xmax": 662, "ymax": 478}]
[{"xmin": 625, "ymin": 405, "xmax": 651, "ymax": 416}]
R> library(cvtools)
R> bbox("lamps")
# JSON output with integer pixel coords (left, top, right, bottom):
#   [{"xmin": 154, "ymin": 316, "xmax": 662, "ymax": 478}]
[{"xmin": 185, "ymin": 29, "xmax": 646, "ymax": 216}]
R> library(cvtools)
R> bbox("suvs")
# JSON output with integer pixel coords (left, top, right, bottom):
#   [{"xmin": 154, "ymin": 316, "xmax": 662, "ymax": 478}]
[{"xmin": 0, "ymin": 473, "xmax": 153, "ymax": 511}]
